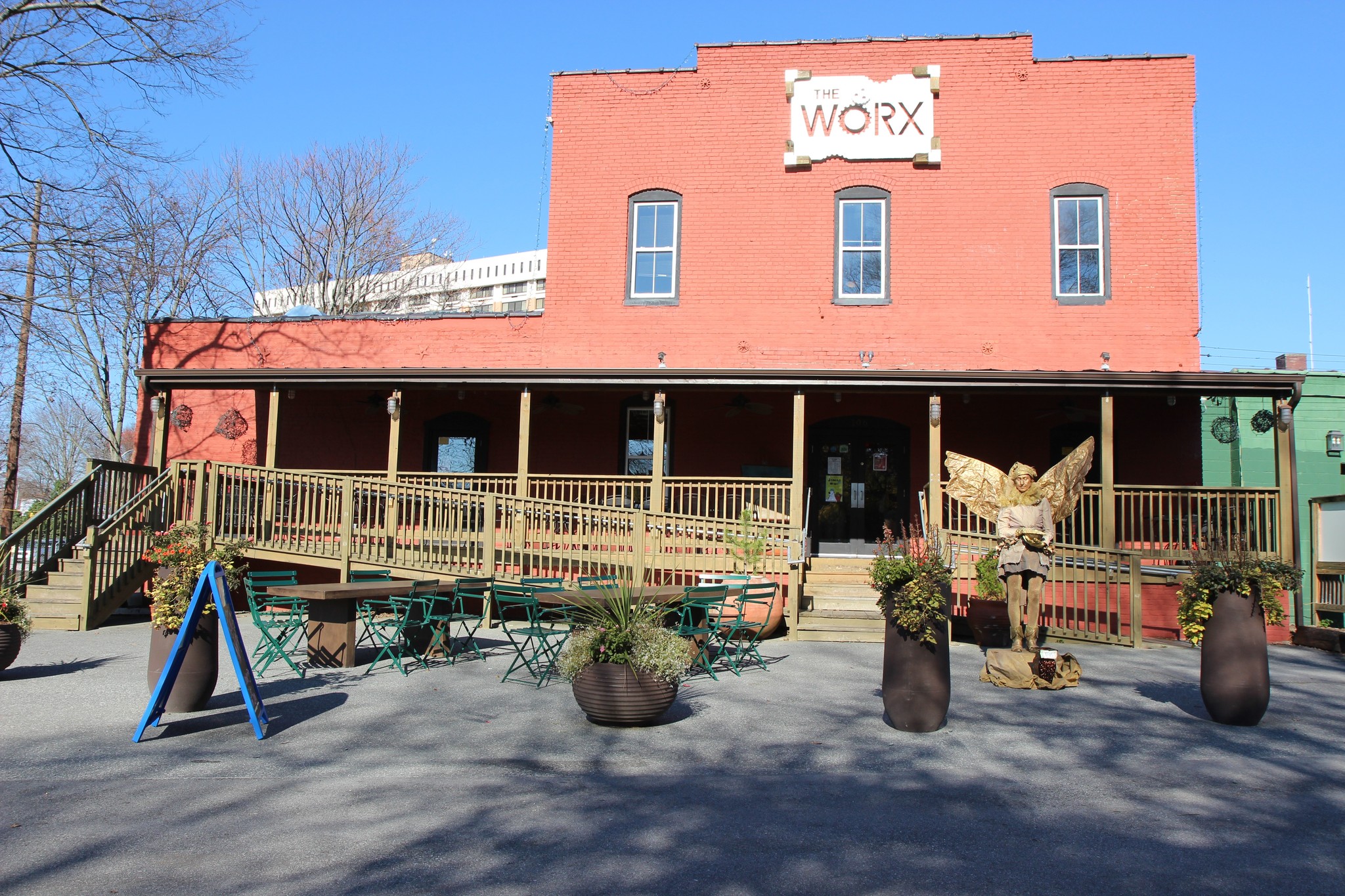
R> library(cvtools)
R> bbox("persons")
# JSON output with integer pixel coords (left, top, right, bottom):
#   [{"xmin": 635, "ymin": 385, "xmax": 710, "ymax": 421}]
[{"xmin": 997, "ymin": 462, "xmax": 1056, "ymax": 654}]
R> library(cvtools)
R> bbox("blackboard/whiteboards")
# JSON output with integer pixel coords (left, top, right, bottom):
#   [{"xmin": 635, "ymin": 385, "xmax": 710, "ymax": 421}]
[{"xmin": 145, "ymin": 561, "xmax": 266, "ymax": 727}]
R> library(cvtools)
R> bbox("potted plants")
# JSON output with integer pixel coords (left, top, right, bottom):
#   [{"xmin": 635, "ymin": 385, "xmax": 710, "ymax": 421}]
[
  {"xmin": 714, "ymin": 508, "xmax": 783, "ymax": 641},
  {"xmin": 966, "ymin": 548, "xmax": 1011, "ymax": 647}
]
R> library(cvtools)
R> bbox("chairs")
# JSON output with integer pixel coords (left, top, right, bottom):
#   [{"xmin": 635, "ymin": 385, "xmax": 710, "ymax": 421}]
[
  {"xmin": 1139, "ymin": 504, "xmax": 1254, "ymax": 567},
  {"xmin": 525, "ymin": 493, "xmax": 790, "ymax": 554},
  {"xmin": 243, "ymin": 567, "xmax": 778, "ymax": 690}
]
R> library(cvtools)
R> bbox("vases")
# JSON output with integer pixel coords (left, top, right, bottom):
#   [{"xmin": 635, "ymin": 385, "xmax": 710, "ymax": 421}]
[
  {"xmin": 0, "ymin": 622, "xmax": 22, "ymax": 671},
  {"xmin": 147, "ymin": 585, "xmax": 219, "ymax": 713},
  {"xmin": 882, "ymin": 582, "xmax": 951, "ymax": 733},
  {"xmin": 1199, "ymin": 578, "xmax": 1270, "ymax": 726},
  {"xmin": 572, "ymin": 661, "xmax": 680, "ymax": 729}
]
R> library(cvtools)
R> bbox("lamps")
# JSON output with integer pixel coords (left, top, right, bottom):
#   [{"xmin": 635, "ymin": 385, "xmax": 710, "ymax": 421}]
[
  {"xmin": 835, "ymin": 393, "xmax": 842, "ymax": 402},
  {"xmin": 1100, "ymin": 351, "xmax": 1111, "ymax": 371},
  {"xmin": 858, "ymin": 350, "xmax": 874, "ymax": 369},
  {"xmin": 642, "ymin": 391, "xmax": 650, "ymax": 401},
  {"xmin": 930, "ymin": 390, "xmax": 941, "ymax": 420},
  {"xmin": 1326, "ymin": 430, "xmax": 1345, "ymax": 452},
  {"xmin": 963, "ymin": 394, "xmax": 971, "ymax": 403},
  {"xmin": 458, "ymin": 390, "xmax": 465, "ymax": 400},
  {"xmin": 653, "ymin": 388, "xmax": 664, "ymax": 418},
  {"xmin": 1278, "ymin": 396, "xmax": 1293, "ymax": 425},
  {"xmin": 658, "ymin": 352, "xmax": 667, "ymax": 368},
  {"xmin": 1167, "ymin": 395, "xmax": 1176, "ymax": 406},
  {"xmin": 150, "ymin": 390, "xmax": 164, "ymax": 413},
  {"xmin": 288, "ymin": 389, "xmax": 296, "ymax": 400},
  {"xmin": 386, "ymin": 390, "xmax": 398, "ymax": 415}
]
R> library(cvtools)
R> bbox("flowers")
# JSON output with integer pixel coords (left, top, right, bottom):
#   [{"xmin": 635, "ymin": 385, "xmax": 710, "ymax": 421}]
[
  {"xmin": 139, "ymin": 517, "xmax": 260, "ymax": 629},
  {"xmin": 552, "ymin": 552, "xmax": 699, "ymax": 690},
  {"xmin": 0, "ymin": 527, "xmax": 49, "ymax": 644},
  {"xmin": 863, "ymin": 513, "xmax": 961, "ymax": 644},
  {"xmin": 1173, "ymin": 532, "xmax": 1306, "ymax": 649}
]
